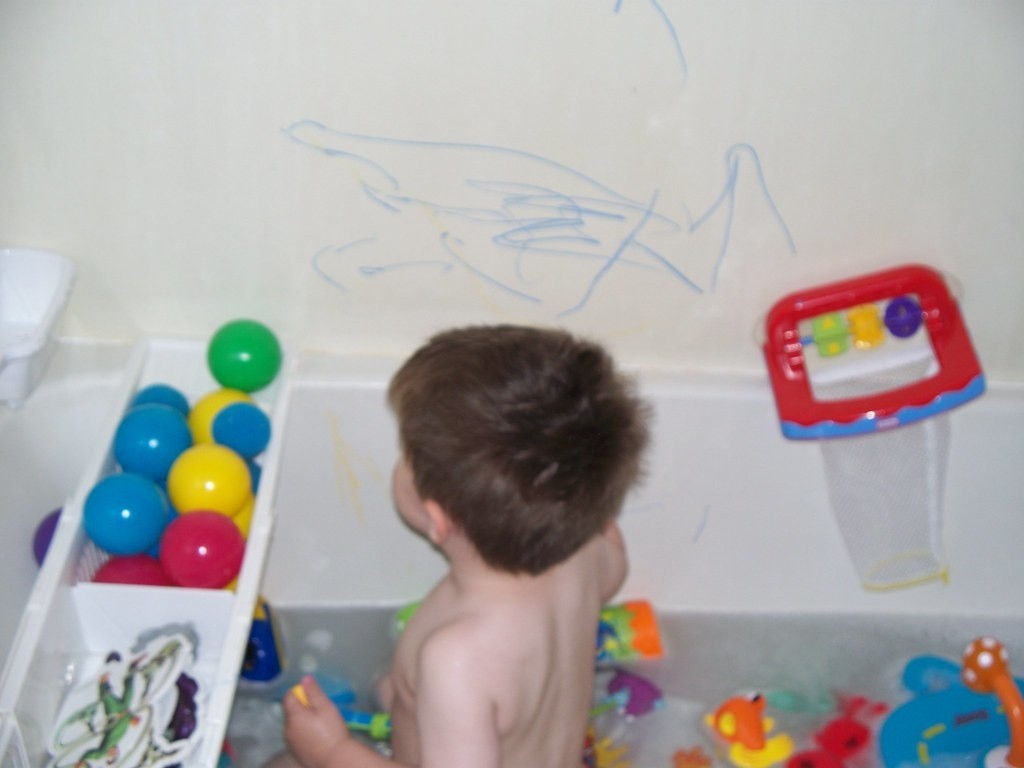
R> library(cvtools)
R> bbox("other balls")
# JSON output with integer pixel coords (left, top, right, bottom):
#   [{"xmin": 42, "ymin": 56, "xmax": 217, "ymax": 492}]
[{"xmin": 82, "ymin": 317, "xmax": 284, "ymax": 588}]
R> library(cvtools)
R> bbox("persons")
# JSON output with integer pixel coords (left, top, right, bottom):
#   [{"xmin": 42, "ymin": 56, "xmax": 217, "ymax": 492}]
[{"xmin": 283, "ymin": 326, "xmax": 647, "ymax": 767}]
